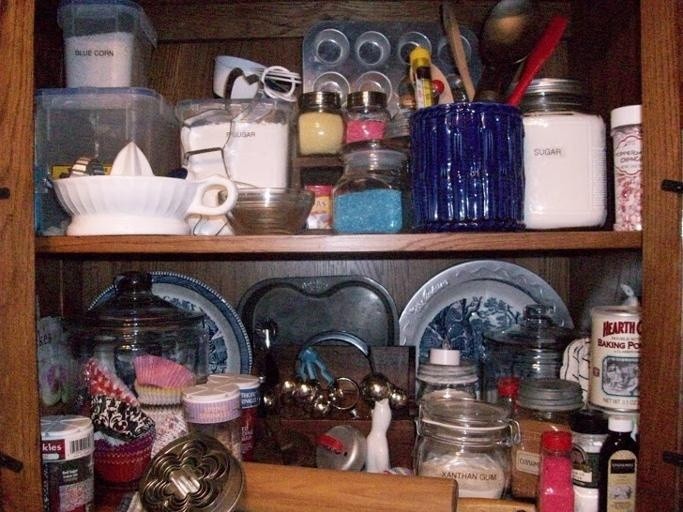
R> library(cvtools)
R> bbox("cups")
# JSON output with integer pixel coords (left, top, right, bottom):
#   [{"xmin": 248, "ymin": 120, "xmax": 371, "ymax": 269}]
[
  {"xmin": 210, "ymin": 55, "xmax": 266, "ymax": 99},
  {"xmin": 51, "ymin": 175, "xmax": 238, "ymax": 236}
]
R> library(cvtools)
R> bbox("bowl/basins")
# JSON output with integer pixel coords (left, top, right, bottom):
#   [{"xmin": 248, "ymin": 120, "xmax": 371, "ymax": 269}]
[{"xmin": 216, "ymin": 186, "xmax": 314, "ymax": 235}]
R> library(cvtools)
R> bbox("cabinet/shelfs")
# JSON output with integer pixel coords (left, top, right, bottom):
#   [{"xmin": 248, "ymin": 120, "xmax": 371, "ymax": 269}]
[{"xmin": 0, "ymin": 1, "xmax": 683, "ymax": 512}]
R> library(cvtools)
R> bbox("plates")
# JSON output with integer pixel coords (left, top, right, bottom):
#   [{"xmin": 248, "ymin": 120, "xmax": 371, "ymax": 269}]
[
  {"xmin": 397, "ymin": 259, "xmax": 576, "ymax": 398},
  {"xmin": 84, "ymin": 270, "xmax": 256, "ymax": 375}
]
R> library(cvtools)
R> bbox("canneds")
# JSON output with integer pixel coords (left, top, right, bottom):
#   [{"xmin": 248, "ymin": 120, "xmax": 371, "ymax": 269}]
[{"xmin": 509, "ymin": 303, "xmax": 641, "ymax": 502}]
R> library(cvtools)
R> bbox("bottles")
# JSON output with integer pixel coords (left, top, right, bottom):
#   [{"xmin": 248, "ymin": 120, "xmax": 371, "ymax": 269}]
[
  {"xmin": 41, "ymin": 372, "xmax": 260, "ymax": 511},
  {"xmin": 409, "ymin": 304, "xmax": 638, "ymax": 512},
  {"xmin": 71, "ymin": 266, "xmax": 210, "ymax": 393},
  {"xmin": 294, "ymin": 46, "xmax": 642, "ymax": 234}
]
